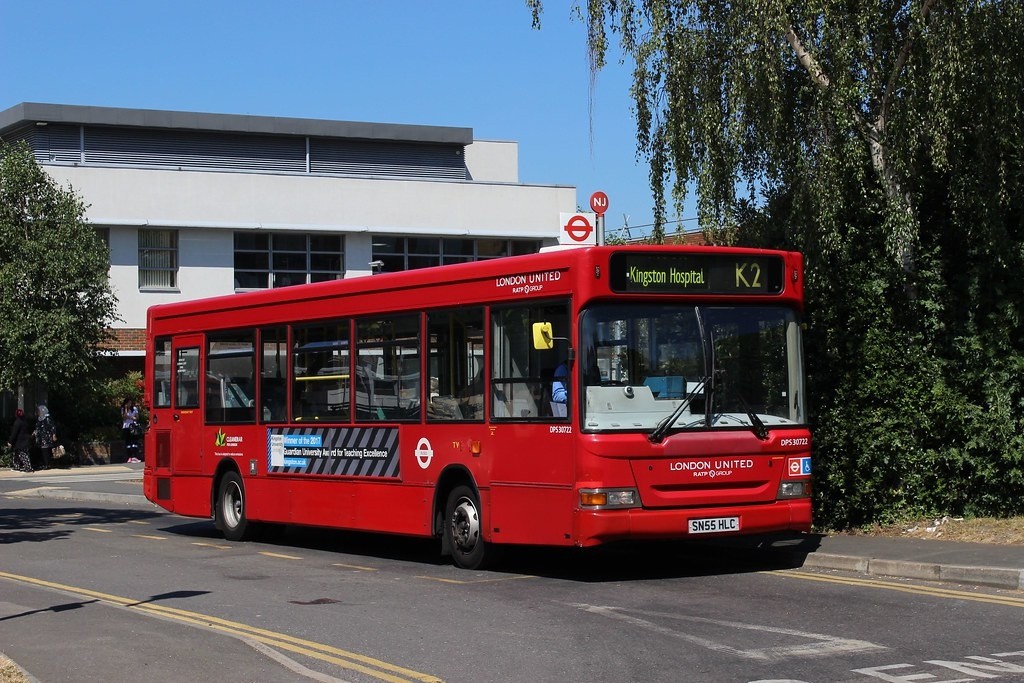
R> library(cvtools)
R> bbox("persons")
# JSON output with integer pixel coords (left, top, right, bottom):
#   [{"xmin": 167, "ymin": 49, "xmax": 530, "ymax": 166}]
[
  {"xmin": 120, "ymin": 398, "xmax": 141, "ymax": 462},
  {"xmin": 6, "ymin": 408, "xmax": 33, "ymax": 472},
  {"xmin": 32, "ymin": 405, "xmax": 56, "ymax": 469},
  {"xmin": 552, "ymin": 344, "xmax": 594, "ymax": 401}
]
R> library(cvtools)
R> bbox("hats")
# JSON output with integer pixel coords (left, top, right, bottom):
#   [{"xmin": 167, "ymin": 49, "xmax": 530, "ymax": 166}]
[{"xmin": 17, "ymin": 409, "xmax": 24, "ymax": 417}]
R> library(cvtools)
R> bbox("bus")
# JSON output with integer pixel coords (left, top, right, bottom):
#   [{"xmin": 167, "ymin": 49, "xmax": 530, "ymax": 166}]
[{"xmin": 142, "ymin": 246, "xmax": 815, "ymax": 568}]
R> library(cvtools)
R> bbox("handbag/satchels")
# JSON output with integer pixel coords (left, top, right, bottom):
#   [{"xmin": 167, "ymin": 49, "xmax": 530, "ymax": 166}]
[
  {"xmin": 52, "ymin": 442, "xmax": 65, "ymax": 458},
  {"xmin": 129, "ymin": 420, "xmax": 144, "ymax": 437}
]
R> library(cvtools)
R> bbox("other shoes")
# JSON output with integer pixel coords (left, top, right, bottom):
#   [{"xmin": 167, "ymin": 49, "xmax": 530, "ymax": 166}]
[
  {"xmin": 42, "ymin": 465, "xmax": 51, "ymax": 470},
  {"xmin": 127, "ymin": 458, "xmax": 132, "ymax": 463},
  {"xmin": 131, "ymin": 457, "xmax": 141, "ymax": 463}
]
